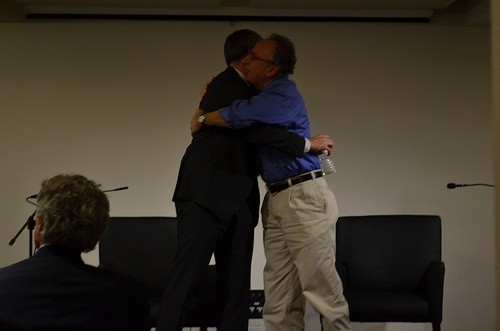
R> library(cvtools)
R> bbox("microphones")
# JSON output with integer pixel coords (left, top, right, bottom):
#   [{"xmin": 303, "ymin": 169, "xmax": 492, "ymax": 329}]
[
  {"xmin": 102, "ymin": 187, "xmax": 128, "ymax": 192},
  {"xmin": 447, "ymin": 183, "xmax": 494, "ymax": 189}
]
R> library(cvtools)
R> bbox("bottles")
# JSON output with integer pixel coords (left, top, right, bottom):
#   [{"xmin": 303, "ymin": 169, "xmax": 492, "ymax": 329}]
[{"xmin": 318, "ymin": 150, "xmax": 336, "ymax": 176}]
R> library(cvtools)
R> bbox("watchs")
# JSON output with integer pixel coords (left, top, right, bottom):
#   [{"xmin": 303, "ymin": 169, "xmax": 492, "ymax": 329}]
[{"xmin": 199, "ymin": 113, "xmax": 206, "ymax": 126}]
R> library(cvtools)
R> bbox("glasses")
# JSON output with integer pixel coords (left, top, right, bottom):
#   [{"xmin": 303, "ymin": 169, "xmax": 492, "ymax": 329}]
[{"xmin": 248, "ymin": 49, "xmax": 279, "ymax": 66}]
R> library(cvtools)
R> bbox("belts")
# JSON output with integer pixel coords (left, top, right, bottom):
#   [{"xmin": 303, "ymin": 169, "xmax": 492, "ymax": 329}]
[{"xmin": 265, "ymin": 170, "xmax": 323, "ymax": 194}]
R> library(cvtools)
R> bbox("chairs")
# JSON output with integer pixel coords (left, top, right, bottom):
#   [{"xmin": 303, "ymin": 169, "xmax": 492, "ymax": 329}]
[
  {"xmin": 331, "ymin": 215, "xmax": 446, "ymax": 331},
  {"xmin": 99, "ymin": 216, "xmax": 202, "ymax": 331}
]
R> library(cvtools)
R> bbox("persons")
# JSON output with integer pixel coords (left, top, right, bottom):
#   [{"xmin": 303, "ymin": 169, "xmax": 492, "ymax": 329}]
[
  {"xmin": 156, "ymin": 28, "xmax": 334, "ymax": 331},
  {"xmin": 190, "ymin": 32, "xmax": 351, "ymax": 331},
  {"xmin": 0, "ymin": 175, "xmax": 151, "ymax": 331}
]
